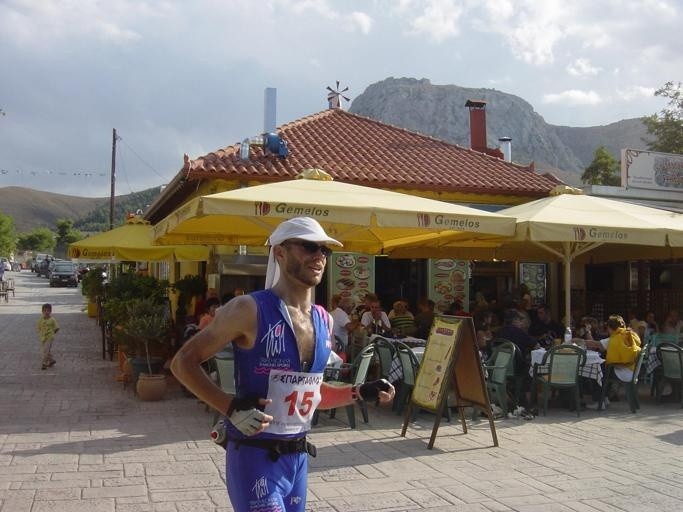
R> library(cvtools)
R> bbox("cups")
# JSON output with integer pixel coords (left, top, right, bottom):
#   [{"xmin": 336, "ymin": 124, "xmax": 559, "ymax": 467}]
[
  {"xmin": 543, "ymin": 333, "xmax": 550, "ymax": 350},
  {"xmin": 553, "ymin": 338, "xmax": 561, "ymax": 346},
  {"xmin": 391, "ymin": 327, "xmax": 397, "ymax": 338}
]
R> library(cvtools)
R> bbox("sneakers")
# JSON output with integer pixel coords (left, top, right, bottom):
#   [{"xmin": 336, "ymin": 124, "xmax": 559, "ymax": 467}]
[{"xmin": 585, "ymin": 397, "xmax": 610, "ymax": 410}]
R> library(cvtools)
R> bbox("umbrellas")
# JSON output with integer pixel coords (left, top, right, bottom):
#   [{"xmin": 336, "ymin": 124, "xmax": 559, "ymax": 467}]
[
  {"xmin": 146, "ymin": 167, "xmax": 517, "ymax": 311},
  {"xmin": 64, "ymin": 212, "xmax": 208, "ymax": 281},
  {"xmin": 478, "ymin": 182, "xmax": 683, "ymax": 333}
]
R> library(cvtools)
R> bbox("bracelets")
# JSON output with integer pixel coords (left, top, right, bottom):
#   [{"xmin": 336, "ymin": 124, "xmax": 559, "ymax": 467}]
[{"xmin": 349, "ymin": 381, "xmax": 365, "ymax": 404}]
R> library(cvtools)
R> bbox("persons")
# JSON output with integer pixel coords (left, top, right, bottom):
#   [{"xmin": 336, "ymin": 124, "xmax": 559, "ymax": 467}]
[
  {"xmin": 35, "ymin": 303, "xmax": 60, "ymax": 371},
  {"xmin": 42, "ymin": 255, "xmax": 107, "ymax": 283},
  {"xmin": 168, "ymin": 215, "xmax": 397, "ymax": 512},
  {"xmin": 164, "ymin": 283, "xmax": 683, "ymax": 420}
]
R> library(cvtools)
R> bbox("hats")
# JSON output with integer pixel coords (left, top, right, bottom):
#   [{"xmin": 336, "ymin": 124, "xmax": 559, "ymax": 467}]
[{"xmin": 268, "ymin": 217, "xmax": 344, "ymax": 249}]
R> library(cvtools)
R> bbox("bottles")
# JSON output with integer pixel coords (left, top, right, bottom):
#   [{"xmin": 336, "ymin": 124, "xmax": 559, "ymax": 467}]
[
  {"xmin": 563, "ymin": 327, "xmax": 572, "ymax": 344},
  {"xmin": 522, "ymin": 272, "xmax": 545, "ymax": 298},
  {"xmin": 376, "ymin": 320, "xmax": 382, "ymax": 336},
  {"xmin": 398, "ymin": 326, "xmax": 400, "ymax": 337},
  {"xmin": 584, "ymin": 330, "xmax": 593, "ymax": 350},
  {"xmin": 370, "ymin": 316, "xmax": 376, "ymax": 334},
  {"xmin": 352, "ymin": 310, "xmax": 358, "ymax": 322}
]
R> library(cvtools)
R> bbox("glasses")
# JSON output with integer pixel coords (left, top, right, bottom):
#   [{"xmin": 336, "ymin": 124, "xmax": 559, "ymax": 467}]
[{"xmin": 286, "ymin": 240, "xmax": 331, "ymax": 256}]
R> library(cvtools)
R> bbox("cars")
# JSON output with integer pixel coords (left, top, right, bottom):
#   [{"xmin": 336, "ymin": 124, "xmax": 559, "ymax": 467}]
[
  {"xmin": 0, "ymin": 256, "xmax": 21, "ymax": 271},
  {"xmin": 30, "ymin": 254, "xmax": 107, "ymax": 279}
]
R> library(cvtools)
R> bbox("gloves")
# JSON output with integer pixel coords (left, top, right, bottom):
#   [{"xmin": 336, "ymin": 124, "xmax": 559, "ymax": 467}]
[
  {"xmin": 358, "ymin": 379, "xmax": 392, "ymax": 402},
  {"xmin": 226, "ymin": 397, "xmax": 265, "ymax": 435}
]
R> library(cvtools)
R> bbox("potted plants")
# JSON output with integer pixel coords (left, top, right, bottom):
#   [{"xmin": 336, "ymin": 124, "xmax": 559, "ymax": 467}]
[{"xmin": 77, "ymin": 267, "xmax": 176, "ymax": 403}]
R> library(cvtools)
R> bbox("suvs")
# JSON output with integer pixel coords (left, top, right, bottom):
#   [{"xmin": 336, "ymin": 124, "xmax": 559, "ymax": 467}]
[{"xmin": 48, "ymin": 262, "xmax": 78, "ymax": 287}]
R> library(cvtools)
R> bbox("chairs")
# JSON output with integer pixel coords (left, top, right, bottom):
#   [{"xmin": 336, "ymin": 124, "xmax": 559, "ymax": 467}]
[
  {"xmin": 0, "ymin": 278, "xmax": 8, "ymax": 302},
  {"xmin": 179, "ymin": 308, "xmax": 682, "ymax": 428},
  {"xmin": 5, "ymin": 277, "xmax": 14, "ymax": 300}
]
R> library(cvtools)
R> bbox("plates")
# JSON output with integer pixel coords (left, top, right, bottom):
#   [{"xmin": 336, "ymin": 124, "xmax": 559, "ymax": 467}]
[
  {"xmin": 433, "ymin": 258, "xmax": 456, "ymax": 272},
  {"xmin": 433, "ymin": 280, "xmax": 451, "ymax": 294},
  {"xmin": 352, "ymin": 266, "xmax": 370, "ymax": 280},
  {"xmin": 334, "ymin": 277, "xmax": 356, "ymax": 292},
  {"xmin": 335, "ymin": 255, "xmax": 356, "ymax": 268},
  {"xmin": 450, "ymin": 268, "xmax": 467, "ymax": 284},
  {"xmin": 352, "ymin": 288, "xmax": 370, "ymax": 301},
  {"xmin": 432, "ymin": 362, "xmax": 443, "ymax": 375}
]
